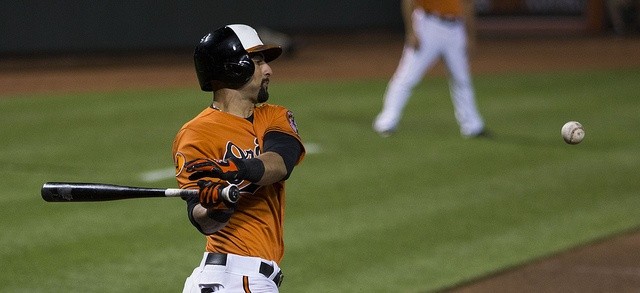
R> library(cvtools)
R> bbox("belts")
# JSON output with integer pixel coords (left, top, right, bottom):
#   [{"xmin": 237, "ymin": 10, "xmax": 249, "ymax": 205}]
[
  {"xmin": 423, "ymin": 10, "xmax": 459, "ymax": 22},
  {"xmin": 205, "ymin": 253, "xmax": 283, "ymax": 286}
]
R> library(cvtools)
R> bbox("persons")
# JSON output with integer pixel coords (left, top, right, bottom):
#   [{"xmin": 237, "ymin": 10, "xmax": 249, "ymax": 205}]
[
  {"xmin": 369, "ymin": 0, "xmax": 497, "ymax": 141},
  {"xmin": 170, "ymin": 22, "xmax": 307, "ymax": 293}
]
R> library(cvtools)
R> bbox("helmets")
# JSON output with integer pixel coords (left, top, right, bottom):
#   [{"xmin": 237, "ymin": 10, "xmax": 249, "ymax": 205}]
[{"xmin": 194, "ymin": 24, "xmax": 282, "ymax": 91}]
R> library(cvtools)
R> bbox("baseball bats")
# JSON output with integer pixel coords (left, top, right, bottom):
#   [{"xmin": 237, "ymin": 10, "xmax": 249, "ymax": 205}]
[{"xmin": 41, "ymin": 182, "xmax": 240, "ymax": 204}]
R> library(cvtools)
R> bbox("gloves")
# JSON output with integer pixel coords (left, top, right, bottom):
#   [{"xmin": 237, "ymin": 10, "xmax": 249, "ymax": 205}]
[
  {"xmin": 197, "ymin": 185, "xmax": 238, "ymax": 223},
  {"xmin": 184, "ymin": 155, "xmax": 264, "ymax": 184}
]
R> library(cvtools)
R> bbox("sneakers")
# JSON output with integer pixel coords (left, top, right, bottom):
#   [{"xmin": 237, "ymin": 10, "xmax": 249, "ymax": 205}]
[
  {"xmin": 476, "ymin": 127, "xmax": 491, "ymax": 139},
  {"xmin": 371, "ymin": 124, "xmax": 396, "ymax": 138}
]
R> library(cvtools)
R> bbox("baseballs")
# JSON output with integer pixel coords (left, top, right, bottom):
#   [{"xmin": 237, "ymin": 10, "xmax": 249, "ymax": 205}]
[{"xmin": 561, "ymin": 121, "xmax": 584, "ymax": 144}]
